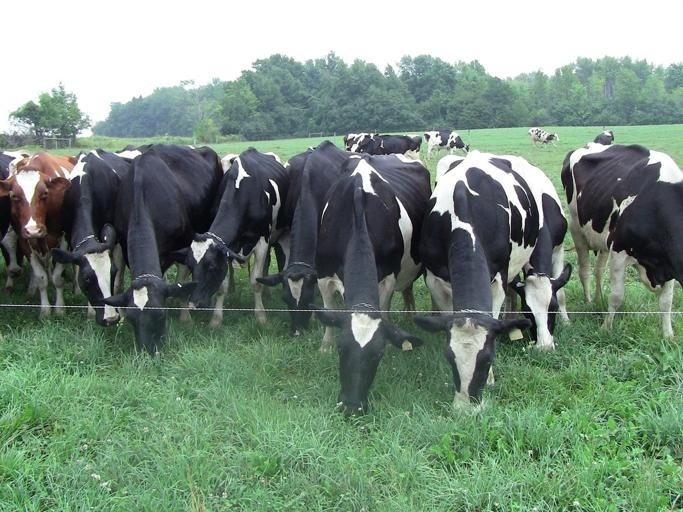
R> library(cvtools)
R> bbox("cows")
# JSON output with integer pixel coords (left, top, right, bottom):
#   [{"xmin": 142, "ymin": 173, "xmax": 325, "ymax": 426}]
[
  {"xmin": 417, "ymin": 150, "xmax": 540, "ymax": 414},
  {"xmin": 528, "ymin": 128, "xmax": 561, "ymax": 151},
  {"xmin": 594, "ymin": 130, "xmax": 615, "ymax": 145},
  {"xmin": 315, "ymin": 152, "xmax": 433, "ymax": 423},
  {"xmin": 343, "ymin": 128, "xmax": 471, "ymax": 161},
  {"xmin": 561, "ymin": 141, "xmax": 683, "ymax": 341},
  {"xmin": 0, "ymin": 139, "xmax": 352, "ymax": 356},
  {"xmin": 495, "ymin": 154, "xmax": 573, "ymax": 350}
]
